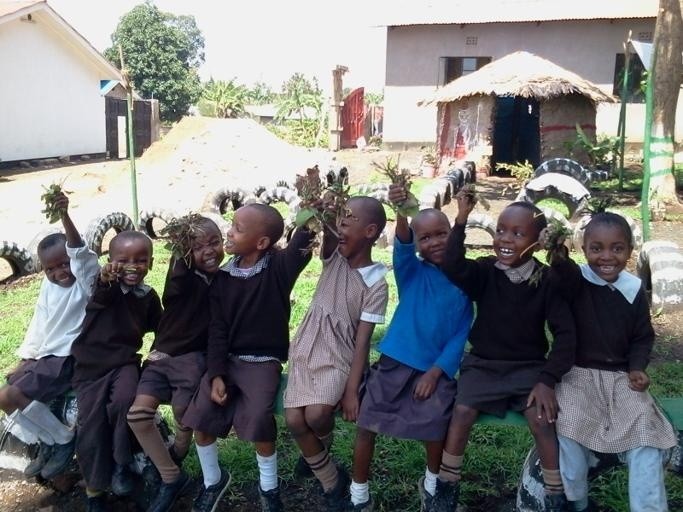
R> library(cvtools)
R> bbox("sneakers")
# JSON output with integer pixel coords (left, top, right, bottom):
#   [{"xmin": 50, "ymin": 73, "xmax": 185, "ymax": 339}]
[
  {"xmin": 84, "ymin": 459, "xmax": 188, "ymax": 511},
  {"xmin": 326, "ymin": 472, "xmax": 374, "ymax": 512},
  {"xmin": 545, "ymin": 492, "xmax": 567, "ymax": 510},
  {"xmin": 294, "ymin": 458, "xmax": 313, "ymax": 479},
  {"xmin": 417, "ymin": 477, "xmax": 457, "ymax": 510},
  {"xmin": 258, "ymin": 483, "xmax": 283, "ymax": 512},
  {"xmin": 193, "ymin": 470, "xmax": 231, "ymax": 512},
  {"xmin": 24, "ymin": 428, "xmax": 76, "ymax": 479}
]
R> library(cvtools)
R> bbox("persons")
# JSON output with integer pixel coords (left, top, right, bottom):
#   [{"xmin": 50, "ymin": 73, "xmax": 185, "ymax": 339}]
[
  {"xmin": 121, "ymin": 212, "xmax": 229, "ymax": 511},
  {"xmin": 173, "ymin": 196, "xmax": 315, "ymax": 511},
  {"xmin": 514, "ymin": 204, "xmax": 679, "ymax": 510},
  {"xmin": 280, "ymin": 185, "xmax": 392, "ymax": 512},
  {"xmin": 340, "ymin": 180, "xmax": 476, "ymax": 509},
  {"xmin": 428, "ymin": 185, "xmax": 569, "ymax": 510},
  {"xmin": 64, "ymin": 229, "xmax": 167, "ymax": 500},
  {"xmin": 0, "ymin": 184, "xmax": 101, "ymax": 485}
]
